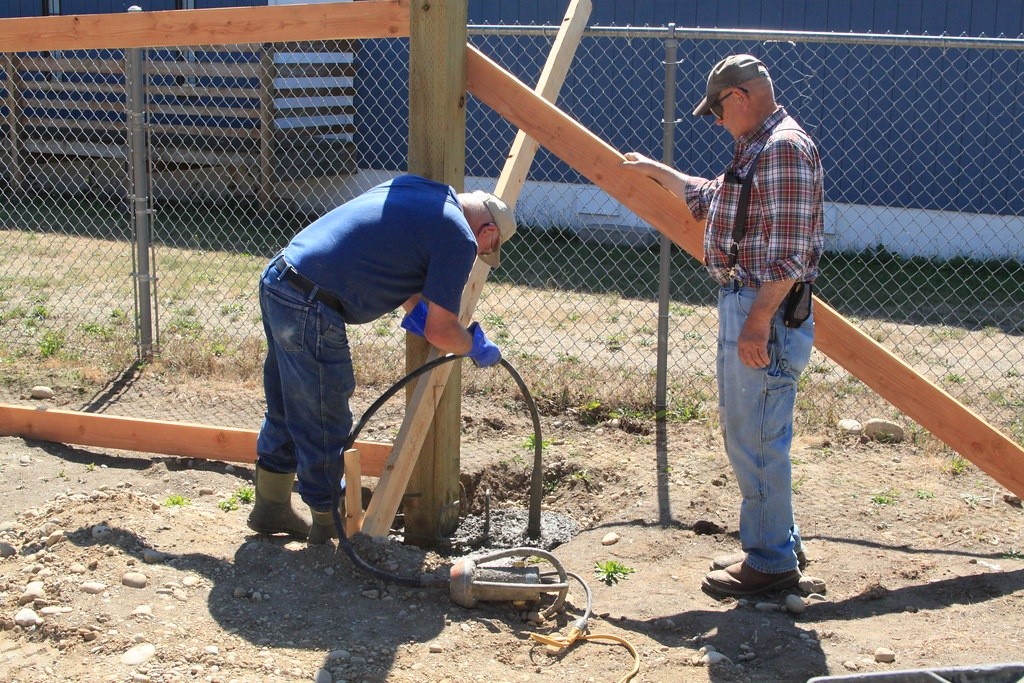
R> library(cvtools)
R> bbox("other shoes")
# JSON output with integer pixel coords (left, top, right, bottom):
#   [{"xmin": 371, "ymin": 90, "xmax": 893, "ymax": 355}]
[
  {"xmin": 710, "ymin": 546, "xmax": 807, "ymax": 571},
  {"xmin": 700, "ymin": 560, "xmax": 802, "ymax": 595}
]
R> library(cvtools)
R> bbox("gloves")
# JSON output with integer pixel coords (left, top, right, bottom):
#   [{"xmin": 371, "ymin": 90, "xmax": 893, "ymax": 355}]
[
  {"xmin": 400, "ymin": 300, "xmax": 428, "ymax": 338},
  {"xmin": 460, "ymin": 321, "xmax": 502, "ymax": 368}
]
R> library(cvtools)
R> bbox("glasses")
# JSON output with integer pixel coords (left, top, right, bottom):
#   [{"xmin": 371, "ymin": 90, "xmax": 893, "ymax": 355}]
[
  {"xmin": 479, "ymin": 226, "xmax": 495, "ymax": 258},
  {"xmin": 709, "ymin": 88, "xmax": 748, "ymax": 120}
]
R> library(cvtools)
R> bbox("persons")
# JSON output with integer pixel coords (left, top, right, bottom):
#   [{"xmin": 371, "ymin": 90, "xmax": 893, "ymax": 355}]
[
  {"xmin": 624, "ymin": 54, "xmax": 826, "ymax": 599},
  {"xmin": 244, "ymin": 174, "xmax": 517, "ymax": 549}
]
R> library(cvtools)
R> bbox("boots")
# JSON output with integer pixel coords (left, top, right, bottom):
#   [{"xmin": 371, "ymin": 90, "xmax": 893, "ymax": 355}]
[
  {"xmin": 247, "ymin": 459, "xmax": 314, "ymax": 540},
  {"xmin": 307, "ymin": 493, "xmax": 355, "ymax": 539}
]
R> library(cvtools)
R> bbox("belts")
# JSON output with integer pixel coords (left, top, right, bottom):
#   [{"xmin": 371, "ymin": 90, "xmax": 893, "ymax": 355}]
[{"xmin": 275, "ymin": 251, "xmax": 343, "ymax": 312}]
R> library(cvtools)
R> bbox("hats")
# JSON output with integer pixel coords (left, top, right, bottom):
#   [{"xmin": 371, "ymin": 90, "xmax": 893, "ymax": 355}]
[
  {"xmin": 693, "ymin": 53, "xmax": 769, "ymax": 116},
  {"xmin": 474, "ymin": 190, "xmax": 516, "ymax": 269}
]
